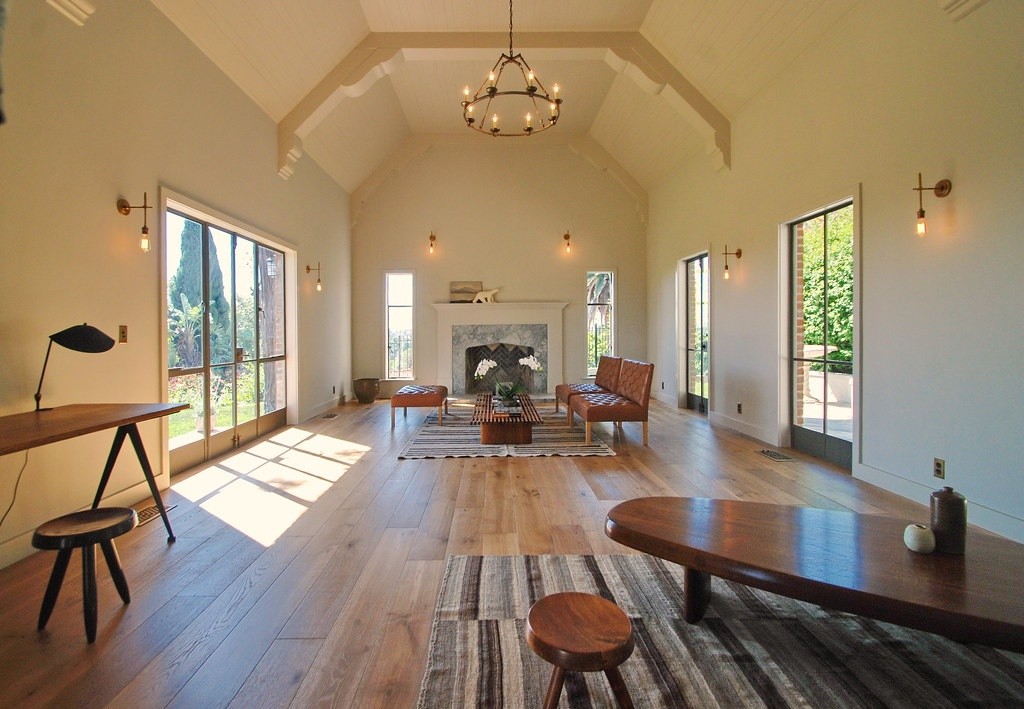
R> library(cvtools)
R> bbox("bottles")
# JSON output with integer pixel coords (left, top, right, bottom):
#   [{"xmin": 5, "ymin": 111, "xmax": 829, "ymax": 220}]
[
  {"xmin": 903, "ymin": 525, "xmax": 935, "ymax": 554},
  {"xmin": 930, "ymin": 486, "xmax": 968, "ymax": 553}
]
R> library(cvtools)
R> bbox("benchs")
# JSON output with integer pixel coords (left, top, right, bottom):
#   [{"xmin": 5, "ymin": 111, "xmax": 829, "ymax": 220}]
[
  {"xmin": 555, "ymin": 356, "xmax": 621, "ymax": 424},
  {"xmin": 569, "ymin": 359, "xmax": 653, "ymax": 443}
]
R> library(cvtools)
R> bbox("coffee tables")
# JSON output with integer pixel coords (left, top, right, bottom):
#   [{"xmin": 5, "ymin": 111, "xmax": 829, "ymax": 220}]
[{"xmin": 470, "ymin": 392, "xmax": 543, "ymax": 445}]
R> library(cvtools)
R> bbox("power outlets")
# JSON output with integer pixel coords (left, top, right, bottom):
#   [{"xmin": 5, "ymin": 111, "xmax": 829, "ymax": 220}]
[
  {"xmin": 933, "ymin": 458, "xmax": 944, "ymax": 479},
  {"xmin": 119, "ymin": 325, "xmax": 127, "ymax": 344}
]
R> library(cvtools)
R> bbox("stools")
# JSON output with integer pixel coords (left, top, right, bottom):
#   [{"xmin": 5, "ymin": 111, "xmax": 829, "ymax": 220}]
[
  {"xmin": 391, "ymin": 385, "xmax": 447, "ymax": 427},
  {"xmin": 31, "ymin": 507, "xmax": 139, "ymax": 644},
  {"xmin": 527, "ymin": 592, "xmax": 636, "ymax": 709}
]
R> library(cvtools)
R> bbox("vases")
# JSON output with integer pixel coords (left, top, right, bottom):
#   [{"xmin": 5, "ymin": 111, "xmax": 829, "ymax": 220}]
[
  {"xmin": 500, "ymin": 398, "xmax": 520, "ymax": 406},
  {"xmin": 354, "ymin": 378, "xmax": 380, "ymax": 403}
]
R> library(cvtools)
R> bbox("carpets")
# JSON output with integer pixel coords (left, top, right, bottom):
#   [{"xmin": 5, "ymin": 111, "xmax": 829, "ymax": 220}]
[
  {"xmin": 398, "ymin": 399, "xmax": 617, "ymax": 459},
  {"xmin": 418, "ymin": 554, "xmax": 1024, "ymax": 709}
]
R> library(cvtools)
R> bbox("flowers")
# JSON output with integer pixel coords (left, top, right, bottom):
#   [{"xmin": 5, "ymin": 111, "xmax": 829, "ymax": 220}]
[{"xmin": 474, "ymin": 354, "xmax": 543, "ymax": 400}]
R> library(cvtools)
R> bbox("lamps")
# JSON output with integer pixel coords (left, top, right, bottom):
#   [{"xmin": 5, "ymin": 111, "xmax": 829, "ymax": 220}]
[
  {"xmin": 306, "ymin": 262, "xmax": 321, "ymax": 290},
  {"xmin": 429, "ymin": 231, "xmax": 436, "ymax": 253},
  {"xmin": 913, "ymin": 173, "xmax": 952, "ymax": 236},
  {"xmin": 117, "ymin": 191, "xmax": 152, "ymax": 251},
  {"xmin": 564, "ymin": 230, "xmax": 571, "ymax": 252},
  {"xmin": 722, "ymin": 244, "xmax": 742, "ymax": 280},
  {"xmin": 461, "ymin": 0, "xmax": 563, "ymax": 138},
  {"xmin": 34, "ymin": 322, "xmax": 115, "ymax": 410}
]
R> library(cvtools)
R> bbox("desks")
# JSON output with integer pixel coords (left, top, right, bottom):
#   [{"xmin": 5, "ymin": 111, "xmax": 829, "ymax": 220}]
[
  {"xmin": 0, "ymin": 402, "xmax": 190, "ymax": 541},
  {"xmin": 606, "ymin": 495, "xmax": 1024, "ymax": 656}
]
